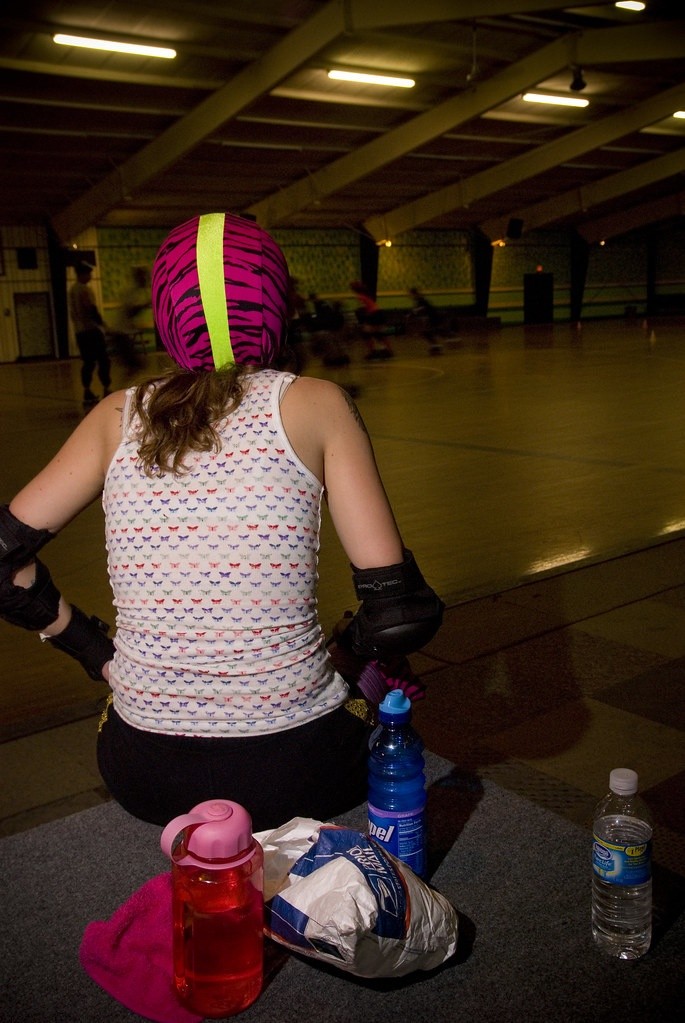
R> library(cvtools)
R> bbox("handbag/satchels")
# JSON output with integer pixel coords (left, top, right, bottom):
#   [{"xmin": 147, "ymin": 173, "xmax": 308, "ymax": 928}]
[{"xmin": 251, "ymin": 817, "xmax": 459, "ymax": 978}]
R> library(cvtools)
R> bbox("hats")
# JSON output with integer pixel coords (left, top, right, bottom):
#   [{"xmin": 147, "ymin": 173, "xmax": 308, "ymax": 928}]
[{"xmin": 150, "ymin": 213, "xmax": 292, "ymax": 370}]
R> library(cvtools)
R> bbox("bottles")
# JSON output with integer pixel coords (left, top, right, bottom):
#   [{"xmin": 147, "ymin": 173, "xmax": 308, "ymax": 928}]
[
  {"xmin": 369, "ymin": 689, "xmax": 427, "ymax": 878},
  {"xmin": 590, "ymin": 768, "xmax": 654, "ymax": 957},
  {"xmin": 161, "ymin": 799, "xmax": 263, "ymax": 1019}
]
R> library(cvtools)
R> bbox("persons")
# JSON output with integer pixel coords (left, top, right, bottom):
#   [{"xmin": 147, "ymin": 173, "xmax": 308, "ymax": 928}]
[
  {"xmin": 0, "ymin": 212, "xmax": 446, "ymax": 832},
  {"xmin": 70, "ymin": 261, "xmax": 112, "ymax": 400},
  {"xmin": 290, "ymin": 279, "xmax": 442, "ymax": 368}
]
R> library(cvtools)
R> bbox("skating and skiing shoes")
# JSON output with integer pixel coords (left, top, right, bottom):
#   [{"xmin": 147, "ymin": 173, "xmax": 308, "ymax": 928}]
[{"xmin": 83, "ymin": 390, "xmax": 99, "ymax": 406}]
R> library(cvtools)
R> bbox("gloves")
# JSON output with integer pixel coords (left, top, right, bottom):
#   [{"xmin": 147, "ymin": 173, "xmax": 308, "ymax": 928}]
[{"xmin": 38, "ymin": 603, "xmax": 116, "ymax": 681}]
